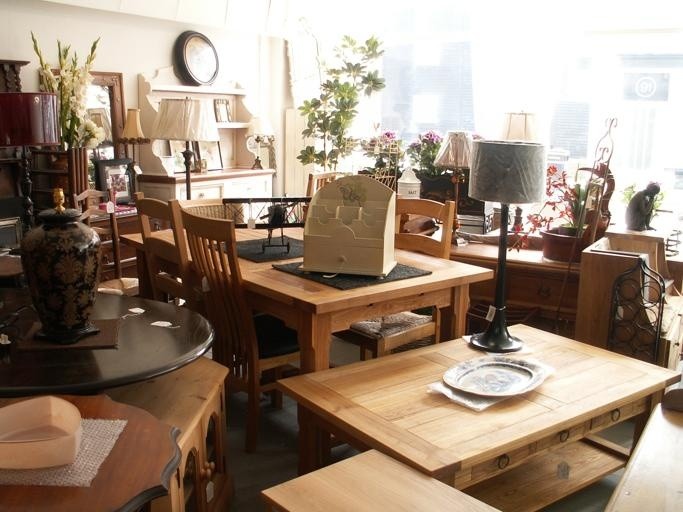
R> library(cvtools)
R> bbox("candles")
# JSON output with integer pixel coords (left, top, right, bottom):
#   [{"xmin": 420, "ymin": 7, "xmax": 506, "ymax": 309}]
[{"xmin": 257, "ymin": 143, "xmax": 260, "ymax": 156}]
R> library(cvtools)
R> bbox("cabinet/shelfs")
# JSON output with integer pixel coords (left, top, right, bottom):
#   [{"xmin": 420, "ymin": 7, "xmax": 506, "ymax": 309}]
[
  {"xmin": 112, "ymin": 356, "xmax": 234, "ymax": 511},
  {"xmin": 581, "ymin": 231, "xmax": 683, "ymax": 372},
  {"xmin": 137, "ymin": 170, "xmax": 274, "ymax": 224}
]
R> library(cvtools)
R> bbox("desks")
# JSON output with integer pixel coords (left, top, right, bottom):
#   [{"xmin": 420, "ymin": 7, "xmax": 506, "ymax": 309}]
[
  {"xmin": 605, "ymin": 402, "xmax": 683, "ymax": 512},
  {"xmin": 450, "ymin": 240, "xmax": 579, "ymax": 339},
  {"xmin": 262, "ymin": 450, "xmax": 500, "ymax": 512}
]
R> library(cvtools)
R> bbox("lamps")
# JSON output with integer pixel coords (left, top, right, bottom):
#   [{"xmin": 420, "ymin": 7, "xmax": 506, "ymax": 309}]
[
  {"xmin": 151, "ymin": 97, "xmax": 221, "ymax": 200},
  {"xmin": 468, "ymin": 139, "xmax": 545, "ymax": 354},
  {"xmin": 0, "ymin": 92, "xmax": 62, "ymax": 256},
  {"xmin": 117, "ymin": 108, "xmax": 150, "ymax": 193},
  {"xmin": 432, "ymin": 131, "xmax": 474, "ymax": 245}
]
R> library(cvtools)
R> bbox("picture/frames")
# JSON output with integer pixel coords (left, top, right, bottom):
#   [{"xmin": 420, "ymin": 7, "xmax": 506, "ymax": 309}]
[
  {"xmin": 168, "ymin": 139, "xmax": 196, "ymax": 174},
  {"xmin": 39, "ymin": 69, "xmax": 131, "ymax": 203},
  {"xmin": 214, "ymin": 98, "xmax": 233, "ymax": 122},
  {"xmin": 196, "ymin": 140, "xmax": 224, "ymax": 171}
]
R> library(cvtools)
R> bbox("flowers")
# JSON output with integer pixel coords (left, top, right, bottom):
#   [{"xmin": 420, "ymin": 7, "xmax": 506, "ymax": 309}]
[
  {"xmin": 408, "ymin": 131, "xmax": 444, "ymax": 174},
  {"xmin": 360, "ymin": 131, "xmax": 400, "ymax": 172},
  {"xmin": 509, "ymin": 165, "xmax": 615, "ymax": 253},
  {"xmin": 31, "ymin": 29, "xmax": 104, "ymax": 149}
]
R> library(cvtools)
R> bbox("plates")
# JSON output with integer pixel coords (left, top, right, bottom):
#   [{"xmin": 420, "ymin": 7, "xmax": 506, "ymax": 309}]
[{"xmin": 442, "ymin": 354, "xmax": 547, "ymax": 398}]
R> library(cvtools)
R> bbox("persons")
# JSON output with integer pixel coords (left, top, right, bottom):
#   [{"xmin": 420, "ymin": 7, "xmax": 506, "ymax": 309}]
[{"xmin": 626, "ymin": 183, "xmax": 660, "ymax": 231}]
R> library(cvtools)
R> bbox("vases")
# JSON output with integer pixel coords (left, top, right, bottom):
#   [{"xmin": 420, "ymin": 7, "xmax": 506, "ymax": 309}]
[
  {"xmin": 68, "ymin": 149, "xmax": 89, "ymax": 225},
  {"xmin": 538, "ymin": 229, "xmax": 589, "ymax": 263}
]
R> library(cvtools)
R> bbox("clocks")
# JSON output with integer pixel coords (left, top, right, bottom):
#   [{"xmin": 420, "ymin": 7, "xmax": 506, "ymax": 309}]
[{"xmin": 174, "ymin": 30, "xmax": 220, "ymax": 86}]
